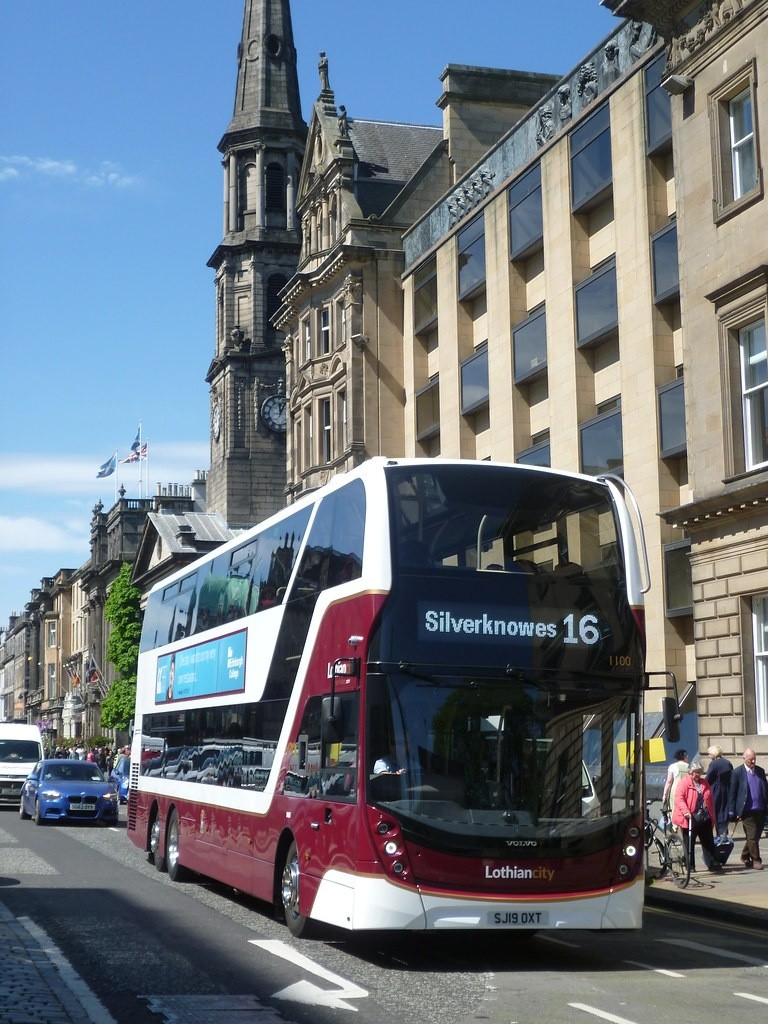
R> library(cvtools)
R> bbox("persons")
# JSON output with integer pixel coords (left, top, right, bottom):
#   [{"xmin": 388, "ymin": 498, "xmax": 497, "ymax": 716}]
[
  {"xmin": 371, "ymin": 729, "xmax": 424, "ymax": 775},
  {"xmin": 670, "ymin": 761, "xmax": 723, "ymax": 873},
  {"xmin": 43, "ymin": 744, "xmax": 130, "ymax": 776},
  {"xmin": 663, "ymin": 749, "xmax": 691, "ymax": 810},
  {"xmin": 704, "ymin": 744, "xmax": 733, "ymax": 836},
  {"xmin": 728, "ymin": 748, "xmax": 768, "ymax": 870}
]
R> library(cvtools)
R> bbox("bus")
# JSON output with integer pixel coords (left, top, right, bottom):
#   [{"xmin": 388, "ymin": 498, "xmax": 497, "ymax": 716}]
[{"xmin": 125, "ymin": 454, "xmax": 685, "ymax": 940}]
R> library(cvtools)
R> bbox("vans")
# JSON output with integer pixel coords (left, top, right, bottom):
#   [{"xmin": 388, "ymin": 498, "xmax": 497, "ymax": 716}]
[{"xmin": 0, "ymin": 723, "xmax": 46, "ymax": 808}]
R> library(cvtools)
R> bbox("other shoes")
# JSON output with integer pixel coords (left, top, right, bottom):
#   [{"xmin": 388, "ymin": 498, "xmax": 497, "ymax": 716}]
[
  {"xmin": 686, "ymin": 863, "xmax": 722, "ymax": 872},
  {"xmin": 741, "ymin": 855, "xmax": 765, "ymax": 871}
]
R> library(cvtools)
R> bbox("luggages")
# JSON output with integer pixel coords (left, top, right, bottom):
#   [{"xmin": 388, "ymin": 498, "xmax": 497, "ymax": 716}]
[{"xmin": 702, "ymin": 815, "xmax": 739, "ymax": 866}]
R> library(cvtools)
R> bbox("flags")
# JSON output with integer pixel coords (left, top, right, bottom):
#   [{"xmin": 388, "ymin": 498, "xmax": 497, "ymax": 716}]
[
  {"xmin": 130, "ymin": 425, "xmax": 140, "ymax": 455},
  {"xmin": 96, "ymin": 452, "xmax": 116, "ymax": 478},
  {"xmin": 65, "ymin": 656, "xmax": 100, "ymax": 687},
  {"xmin": 117, "ymin": 441, "xmax": 147, "ymax": 464}
]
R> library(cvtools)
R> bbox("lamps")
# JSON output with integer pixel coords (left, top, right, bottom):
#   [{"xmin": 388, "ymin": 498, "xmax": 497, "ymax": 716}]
[{"xmin": 659, "ymin": 75, "xmax": 694, "ymax": 96}]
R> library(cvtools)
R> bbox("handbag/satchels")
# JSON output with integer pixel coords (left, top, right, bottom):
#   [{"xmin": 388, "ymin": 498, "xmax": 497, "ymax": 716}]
[
  {"xmin": 658, "ymin": 803, "xmax": 672, "ymax": 832},
  {"xmin": 690, "ymin": 810, "xmax": 709, "ymax": 828}
]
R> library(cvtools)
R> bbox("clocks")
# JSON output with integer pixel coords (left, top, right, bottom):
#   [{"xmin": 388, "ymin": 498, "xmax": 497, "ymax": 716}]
[{"xmin": 260, "ymin": 394, "xmax": 286, "ymax": 433}]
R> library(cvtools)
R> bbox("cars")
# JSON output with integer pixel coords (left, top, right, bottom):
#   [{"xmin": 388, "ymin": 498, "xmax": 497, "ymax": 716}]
[
  {"xmin": 140, "ymin": 734, "xmax": 355, "ymax": 799},
  {"xmin": 19, "ymin": 759, "xmax": 120, "ymax": 827}
]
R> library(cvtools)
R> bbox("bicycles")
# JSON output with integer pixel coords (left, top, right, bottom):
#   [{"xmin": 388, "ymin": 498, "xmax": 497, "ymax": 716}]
[{"xmin": 645, "ymin": 797, "xmax": 690, "ymax": 889}]
